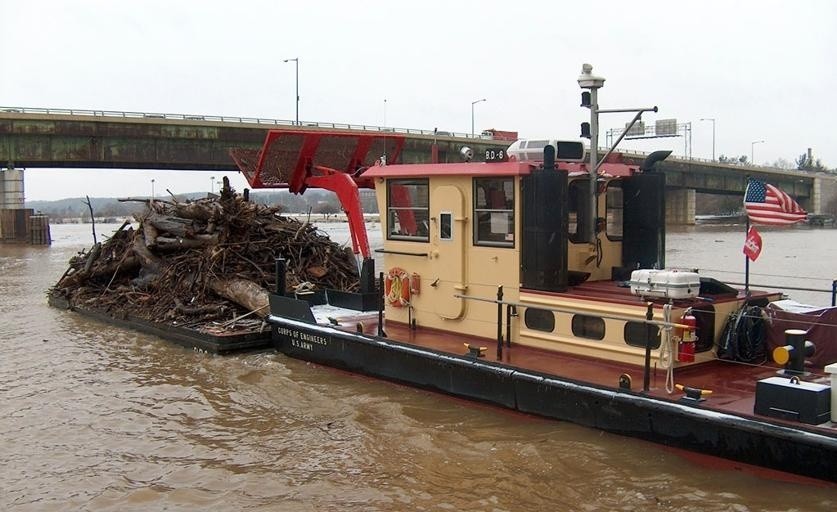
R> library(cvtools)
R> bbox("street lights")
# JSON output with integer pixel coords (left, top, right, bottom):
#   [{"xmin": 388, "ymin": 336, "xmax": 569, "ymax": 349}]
[
  {"xmin": 210, "ymin": 176, "xmax": 215, "ymax": 194},
  {"xmin": 383, "ymin": 98, "xmax": 387, "ymax": 129},
  {"xmin": 282, "ymin": 58, "xmax": 299, "ymax": 125},
  {"xmin": 470, "ymin": 99, "xmax": 486, "ymax": 138},
  {"xmin": 150, "ymin": 179, "xmax": 155, "ymax": 200},
  {"xmin": 750, "ymin": 140, "xmax": 764, "ymax": 166},
  {"xmin": 699, "ymin": 117, "xmax": 716, "ymax": 163}
]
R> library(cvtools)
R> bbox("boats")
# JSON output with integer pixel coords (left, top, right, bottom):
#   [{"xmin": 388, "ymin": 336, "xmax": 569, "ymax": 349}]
[{"xmin": 225, "ymin": 64, "xmax": 836, "ymax": 483}]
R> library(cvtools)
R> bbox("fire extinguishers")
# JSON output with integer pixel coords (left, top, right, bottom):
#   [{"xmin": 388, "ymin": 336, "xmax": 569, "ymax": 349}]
[{"xmin": 678, "ymin": 306, "xmax": 696, "ymax": 362}]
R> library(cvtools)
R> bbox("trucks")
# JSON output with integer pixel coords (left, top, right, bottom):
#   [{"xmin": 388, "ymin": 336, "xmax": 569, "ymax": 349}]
[{"xmin": 478, "ymin": 129, "xmax": 518, "ymax": 141}]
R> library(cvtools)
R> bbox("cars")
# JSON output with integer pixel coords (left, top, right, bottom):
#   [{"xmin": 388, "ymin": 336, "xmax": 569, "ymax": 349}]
[{"xmin": 437, "ymin": 132, "xmax": 451, "ymax": 136}]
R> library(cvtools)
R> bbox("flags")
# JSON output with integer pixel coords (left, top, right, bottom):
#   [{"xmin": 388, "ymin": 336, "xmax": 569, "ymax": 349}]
[{"xmin": 743, "ymin": 178, "xmax": 809, "ymax": 228}]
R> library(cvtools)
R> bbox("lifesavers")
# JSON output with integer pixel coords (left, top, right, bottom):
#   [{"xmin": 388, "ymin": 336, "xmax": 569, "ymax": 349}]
[{"xmin": 385, "ymin": 268, "xmax": 410, "ymax": 307}]
